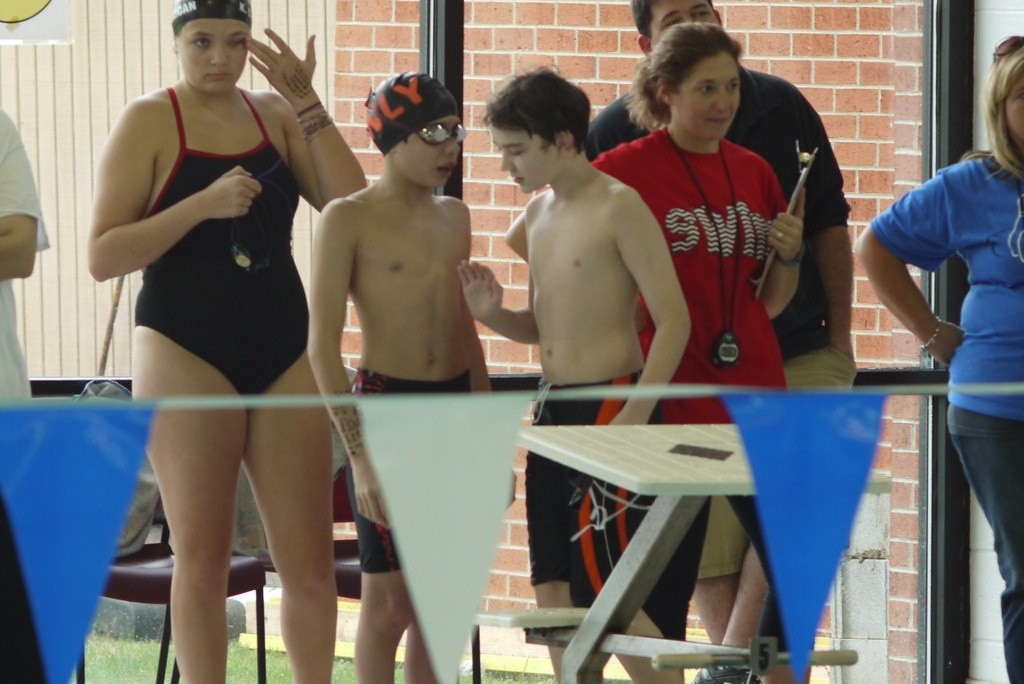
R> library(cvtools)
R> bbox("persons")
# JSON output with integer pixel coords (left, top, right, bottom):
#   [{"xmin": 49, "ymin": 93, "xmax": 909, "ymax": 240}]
[
  {"xmin": 306, "ymin": 71, "xmax": 493, "ymax": 684},
  {"xmin": 85, "ymin": 0, "xmax": 369, "ymax": 684},
  {"xmin": 583, "ymin": 0, "xmax": 853, "ymax": 684},
  {"xmin": 0, "ymin": 105, "xmax": 52, "ymax": 407},
  {"xmin": 853, "ymin": 36, "xmax": 1024, "ymax": 684},
  {"xmin": 454, "ymin": 65, "xmax": 693, "ymax": 684}
]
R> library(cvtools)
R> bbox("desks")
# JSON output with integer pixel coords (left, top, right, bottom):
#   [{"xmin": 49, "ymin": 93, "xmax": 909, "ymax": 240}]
[{"xmin": 510, "ymin": 417, "xmax": 822, "ymax": 683}]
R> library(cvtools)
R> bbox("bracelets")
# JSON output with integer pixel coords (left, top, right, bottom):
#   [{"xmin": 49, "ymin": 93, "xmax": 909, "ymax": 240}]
[
  {"xmin": 296, "ymin": 102, "xmax": 339, "ymax": 143},
  {"xmin": 915, "ymin": 314, "xmax": 942, "ymax": 353}
]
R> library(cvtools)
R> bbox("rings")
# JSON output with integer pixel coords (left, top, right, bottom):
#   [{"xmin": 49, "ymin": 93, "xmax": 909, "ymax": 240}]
[{"xmin": 776, "ymin": 232, "xmax": 786, "ymax": 242}]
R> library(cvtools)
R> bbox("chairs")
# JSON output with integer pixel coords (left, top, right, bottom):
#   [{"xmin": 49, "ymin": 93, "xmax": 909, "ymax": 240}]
[{"xmin": 31, "ymin": 375, "xmax": 481, "ymax": 684}]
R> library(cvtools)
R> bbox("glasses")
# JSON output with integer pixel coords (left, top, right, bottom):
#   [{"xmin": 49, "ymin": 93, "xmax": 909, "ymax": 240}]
[
  {"xmin": 410, "ymin": 123, "xmax": 469, "ymax": 146},
  {"xmin": 993, "ymin": 35, "xmax": 1023, "ymax": 63},
  {"xmin": 233, "ymin": 242, "xmax": 276, "ymax": 275}
]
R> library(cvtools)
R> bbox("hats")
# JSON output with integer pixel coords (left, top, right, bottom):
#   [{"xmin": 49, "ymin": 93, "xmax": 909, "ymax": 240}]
[
  {"xmin": 172, "ymin": 0, "xmax": 253, "ymax": 35},
  {"xmin": 367, "ymin": 72, "xmax": 458, "ymax": 154}
]
